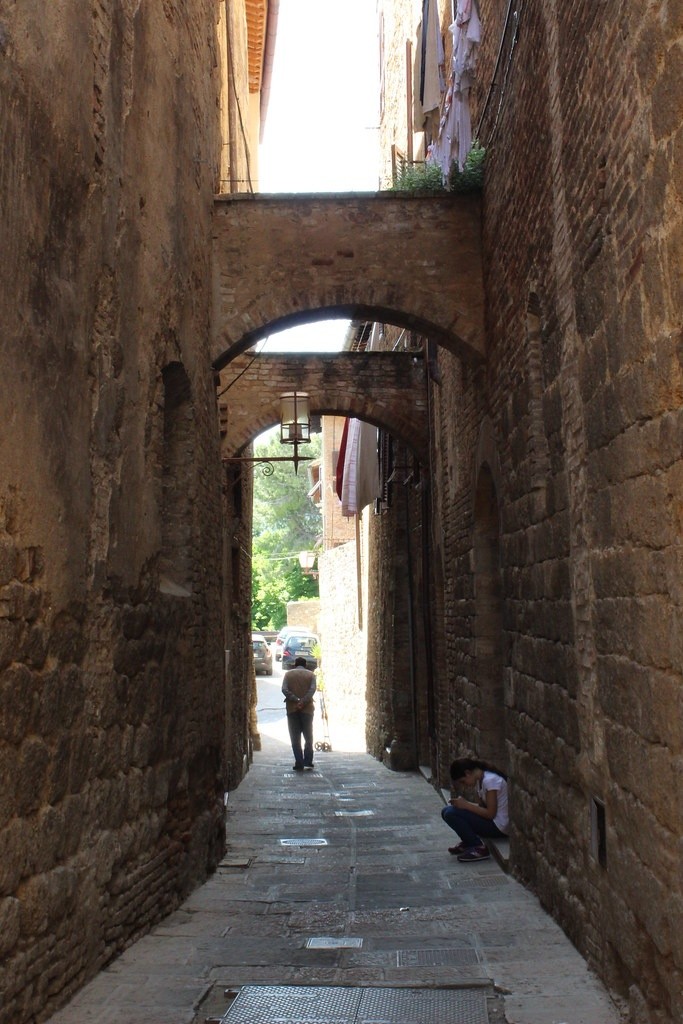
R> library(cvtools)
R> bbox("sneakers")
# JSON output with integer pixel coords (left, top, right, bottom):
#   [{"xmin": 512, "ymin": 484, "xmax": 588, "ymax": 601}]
[
  {"xmin": 457, "ymin": 844, "xmax": 490, "ymax": 861},
  {"xmin": 448, "ymin": 841, "xmax": 486, "ymax": 855}
]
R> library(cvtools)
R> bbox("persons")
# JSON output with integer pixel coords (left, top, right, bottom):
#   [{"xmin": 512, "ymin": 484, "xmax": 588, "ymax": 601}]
[
  {"xmin": 440, "ymin": 757, "xmax": 509, "ymax": 862},
  {"xmin": 280, "ymin": 656, "xmax": 317, "ymax": 771}
]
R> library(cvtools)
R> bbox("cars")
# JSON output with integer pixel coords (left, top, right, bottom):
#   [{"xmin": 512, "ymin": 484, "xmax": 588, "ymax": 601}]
[{"xmin": 283, "ymin": 635, "xmax": 317, "ymax": 670}]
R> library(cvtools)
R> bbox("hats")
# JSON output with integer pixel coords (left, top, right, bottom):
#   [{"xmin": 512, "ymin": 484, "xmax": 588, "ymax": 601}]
[{"xmin": 295, "ymin": 657, "xmax": 306, "ymax": 666}]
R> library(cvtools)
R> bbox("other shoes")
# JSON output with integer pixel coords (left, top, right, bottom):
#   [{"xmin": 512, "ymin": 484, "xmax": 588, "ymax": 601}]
[
  {"xmin": 304, "ymin": 762, "xmax": 314, "ymax": 767},
  {"xmin": 293, "ymin": 767, "xmax": 304, "ymax": 771}
]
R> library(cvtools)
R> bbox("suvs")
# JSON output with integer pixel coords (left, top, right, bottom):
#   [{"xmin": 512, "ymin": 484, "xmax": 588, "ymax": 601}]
[
  {"xmin": 252, "ymin": 635, "xmax": 273, "ymax": 675},
  {"xmin": 275, "ymin": 621, "xmax": 330, "ymax": 662}
]
